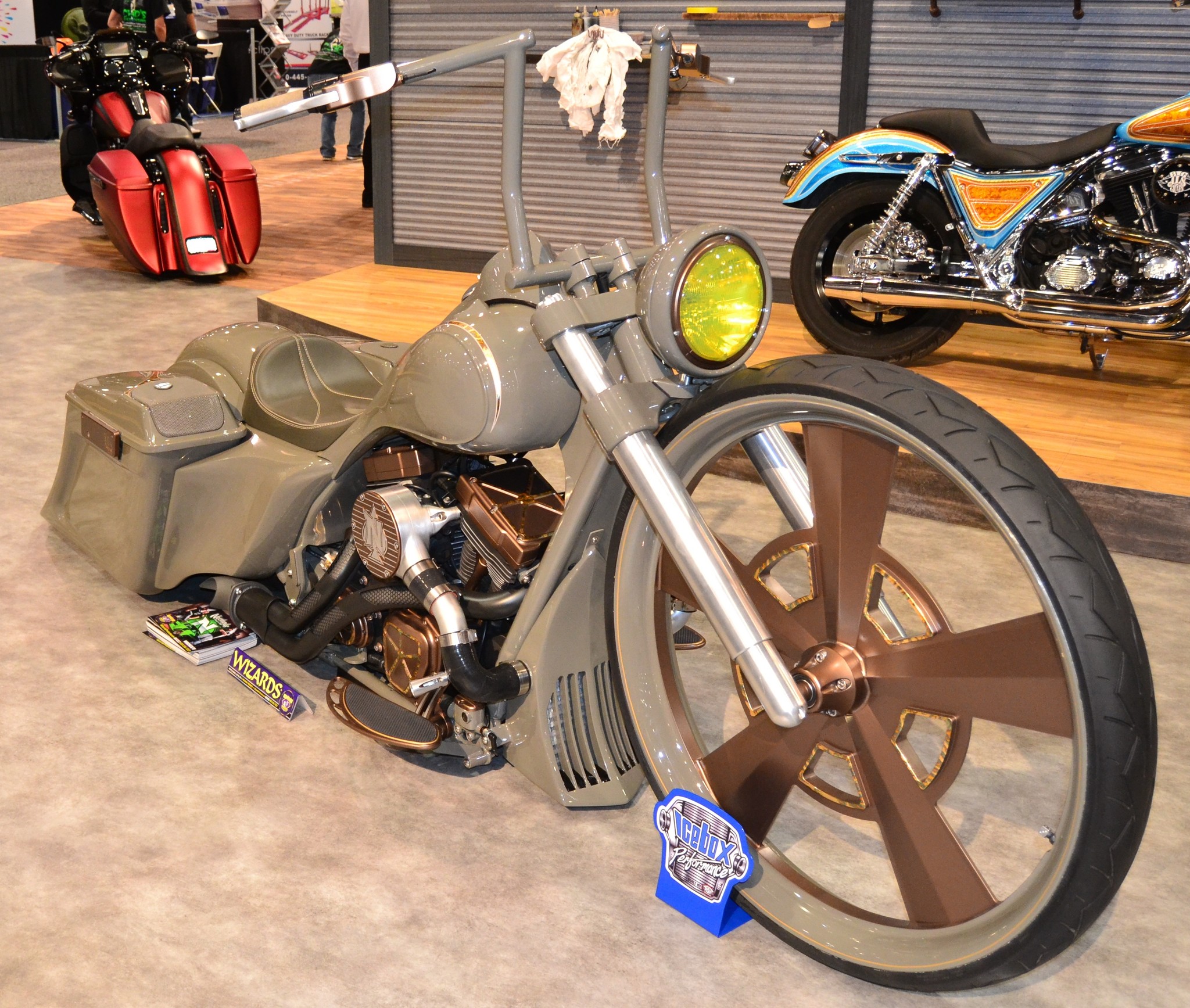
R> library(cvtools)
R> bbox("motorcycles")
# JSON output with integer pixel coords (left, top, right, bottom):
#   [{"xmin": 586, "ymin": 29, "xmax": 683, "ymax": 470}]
[
  {"xmin": 34, "ymin": 28, "xmax": 261, "ymax": 279},
  {"xmin": 779, "ymin": 83, "xmax": 1189, "ymax": 367},
  {"xmin": 36, "ymin": 26, "xmax": 1164, "ymax": 992}
]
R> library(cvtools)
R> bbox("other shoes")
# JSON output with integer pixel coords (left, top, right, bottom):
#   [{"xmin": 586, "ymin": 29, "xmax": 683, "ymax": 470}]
[
  {"xmin": 321, "ymin": 155, "xmax": 335, "ymax": 160},
  {"xmin": 345, "ymin": 150, "xmax": 363, "ymax": 161}
]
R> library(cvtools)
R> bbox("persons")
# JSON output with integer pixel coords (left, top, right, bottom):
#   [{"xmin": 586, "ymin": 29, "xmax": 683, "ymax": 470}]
[
  {"xmin": 305, "ymin": 26, "xmax": 365, "ymax": 161},
  {"xmin": 340, "ymin": 0, "xmax": 374, "ymax": 209},
  {"xmin": 83, "ymin": 0, "xmax": 210, "ymax": 138}
]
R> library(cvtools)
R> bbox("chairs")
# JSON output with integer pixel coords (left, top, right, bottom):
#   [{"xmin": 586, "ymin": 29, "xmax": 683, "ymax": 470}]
[{"xmin": 187, "ymin": 42, "xmax": 223, "ymax": 118}]
